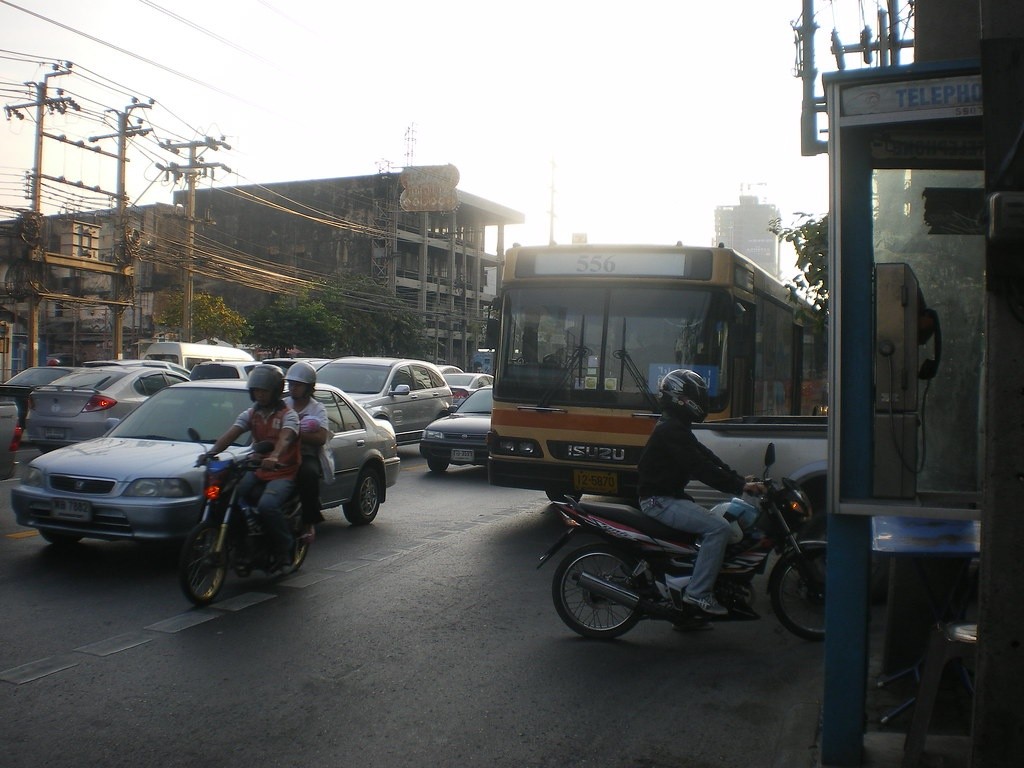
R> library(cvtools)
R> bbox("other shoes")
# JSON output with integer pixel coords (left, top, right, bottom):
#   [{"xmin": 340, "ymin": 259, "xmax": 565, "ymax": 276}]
[{"xmin": 301, "ymin": 523, "xmax": 317, "ymax": 543}]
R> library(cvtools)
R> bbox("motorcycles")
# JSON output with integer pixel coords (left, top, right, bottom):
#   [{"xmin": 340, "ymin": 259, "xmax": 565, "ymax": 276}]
[
  {"xmin": 178, "ymin": 430, "xmax": 316, "ymax": 605},
  {"xmin": 532, "ymin": 439, "xmax": 830, "ymax": 647}
]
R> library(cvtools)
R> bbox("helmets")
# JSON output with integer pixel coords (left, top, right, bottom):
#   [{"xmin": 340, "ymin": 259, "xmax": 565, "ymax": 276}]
[
  {"xmin": 282, "ymin": 362, "xmax": 317, "ymax": 384},
  {"xmin": 659, "ymin": 369, "xmax": 709, "ymax": 423},
  {"xmin": 247, "ymin": 364, "xmax": 284, "ymax": 404},
  {"xmin": 710, "ymin": 497, "xmax": 763, "ymax": 546}
]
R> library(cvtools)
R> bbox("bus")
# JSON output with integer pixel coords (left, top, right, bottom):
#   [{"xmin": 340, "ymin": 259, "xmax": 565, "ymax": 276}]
[{"xmin": 484, "ymin": 232, "xmax": 830, "ymax": 492}]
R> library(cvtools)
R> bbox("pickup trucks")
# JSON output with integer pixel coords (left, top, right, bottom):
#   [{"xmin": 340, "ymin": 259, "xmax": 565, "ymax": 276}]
[{"xmin": 0, "ymin": 400, "xmax": 21, "ymax": 486}]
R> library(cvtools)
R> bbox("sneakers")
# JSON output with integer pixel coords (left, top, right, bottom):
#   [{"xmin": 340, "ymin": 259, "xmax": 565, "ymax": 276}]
[
  {"xmin": 682, "ymin": 591, "xmax": 728, "ymax": 614},
  {"xmin": 281, "ymin": 538, "xmax": 300, "ymax": 575}
]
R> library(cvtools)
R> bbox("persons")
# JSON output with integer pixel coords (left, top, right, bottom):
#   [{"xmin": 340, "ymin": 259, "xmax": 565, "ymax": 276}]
[
  {"xmin": 280, "ymin": 361, "xmax": 334, "ymax": 543},
  {"xmin": 196, "ymin": 364, "xmax": 300, "ymax": 577},
  {"xmin": 636, "ymin": 369, "xmax": 769, "ymax": 630},
  {"xmin": 544, "ymin": 326, "xmax": 593, "ymax": 387}
]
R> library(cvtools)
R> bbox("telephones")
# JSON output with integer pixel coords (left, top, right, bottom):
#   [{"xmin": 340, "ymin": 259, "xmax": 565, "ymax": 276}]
[{"xmin": 876, "ymin": 261, "xmax": 944, "ymax": 414}]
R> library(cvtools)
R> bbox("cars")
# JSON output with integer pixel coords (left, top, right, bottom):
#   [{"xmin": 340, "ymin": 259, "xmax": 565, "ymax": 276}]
[
  {"xmin": 23, "ymin": 367, "xmax": 191, "ymax": 446},
  {"xmin": 82, "ymin": 357, "xmax": 191, "ymax": 380},
  {"xmin": 263, "ymin": 356, "xmax": 332, "ymax": 378},
  {"xmin": 10, "ymin": 376, "xmax": 400, "ymax": 553},
  {"xmin": 191, "ymin": 360, "xmax": 261, "ymax": 380},
  {"xmin": 441, "ymin": 372, "xmax": 495, "ymax": 412},
  {"xmin": 313, "ymin": 356, "xmax": 454, "ymax": 446},
  {"xmin": 0, "ymin": 366, "xmax": 73, "ymax": 430},
  {"xmin": 436, "ymin": 363, "xmax": 464, "ymax": 373},
  {"xmin": 417, "ymin": 385, "xmax": 494, "ymax": 472}
]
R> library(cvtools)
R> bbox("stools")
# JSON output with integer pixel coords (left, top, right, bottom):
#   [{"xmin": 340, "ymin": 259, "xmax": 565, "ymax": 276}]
[{"xmin": 899, "ymin": 617, "xmax": 978, "ymax": 768}]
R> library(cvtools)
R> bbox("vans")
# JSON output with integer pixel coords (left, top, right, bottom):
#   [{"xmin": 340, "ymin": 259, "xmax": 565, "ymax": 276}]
[{"xmin": 138, "ymin": 341, "xmax": 255, "ymax": 371}]
